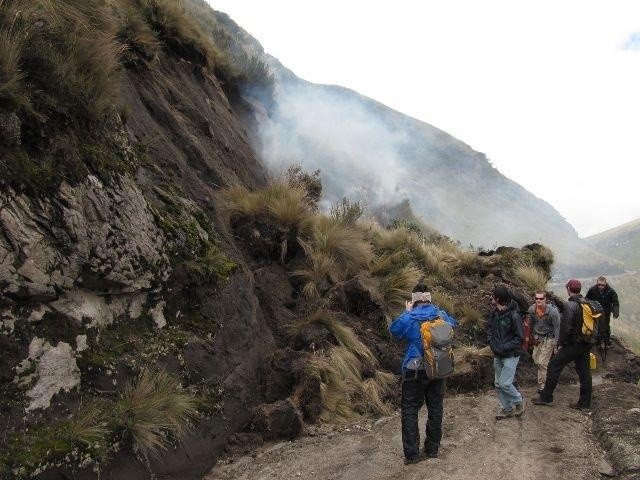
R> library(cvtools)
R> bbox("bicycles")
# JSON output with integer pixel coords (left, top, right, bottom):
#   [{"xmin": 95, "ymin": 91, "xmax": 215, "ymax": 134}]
[{"xmin": 596, "ymin": 313, "xmax": 610, "ymax": 362}]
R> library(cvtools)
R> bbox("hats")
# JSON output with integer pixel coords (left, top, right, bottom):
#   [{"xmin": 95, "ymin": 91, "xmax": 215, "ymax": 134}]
[{"xmin": 565, "ymin": 280, "xmax": 581, "ymax": 289}]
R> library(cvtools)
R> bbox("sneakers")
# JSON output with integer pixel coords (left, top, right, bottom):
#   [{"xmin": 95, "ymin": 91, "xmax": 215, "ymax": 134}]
[
  {"xmin": 533, "ymin": 397, "xmax": 554, "ymax": 406},
  {"xmin": 495, "ymin": 406, "xmax": 514, "ymax": 420},
  {"xmin": 514, "ymin": 403, "xmax": 523, "ymax": 418},
  {"xmin": 569, "ymin": 402, "xmax": 590, "ymax": 412},
  {"xmin": 404, "ymin": 455, "xmax": 421, "ymax": 465},
  {"xmin": 424, "ymin": 449, "xmax": 438, "ymax": 459}
]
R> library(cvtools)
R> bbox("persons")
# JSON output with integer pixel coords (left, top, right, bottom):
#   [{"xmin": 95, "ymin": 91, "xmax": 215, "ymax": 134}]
[
  {"xmin": 390, "ymin": 284, "xmax": 459, "ymax": 464},
  {"xmin": 531, "ymin": 278, "xmax": 599, "ymax": 413},
  {"xmin": 523, "ymin": 290, "xmax": 562, "ymax": 393},
  {"xmin": 485, "ymin": 288, "xmax": 528, "ymax": 420},
  {"xmin": 584, "ymin": 277, "xmax": 619, "ymax": 352}
]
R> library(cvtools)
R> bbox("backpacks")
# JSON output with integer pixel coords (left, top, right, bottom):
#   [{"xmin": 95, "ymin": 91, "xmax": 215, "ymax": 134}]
[
  {"xmin": 417, "ymin": 314, "xmax": 458, "ymax": 380},
  {"xmin": 576, "ymin": 297, "xmax": 605, "ymax": 336}
]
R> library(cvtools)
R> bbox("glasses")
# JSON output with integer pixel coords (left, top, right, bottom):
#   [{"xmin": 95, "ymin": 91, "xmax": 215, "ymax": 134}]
[
  {"xmin": 597, "ymin": 283, "xmax": 605, "ymax": 286},
  {"xmin": 534, "ymin": 297, "xmax": 544, "ymax": 300}
]
